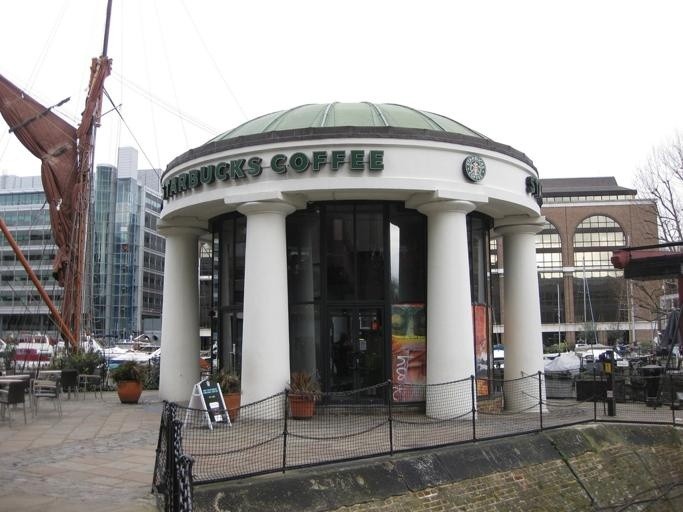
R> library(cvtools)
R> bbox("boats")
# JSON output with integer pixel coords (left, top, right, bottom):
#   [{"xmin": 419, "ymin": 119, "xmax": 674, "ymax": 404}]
[
  {"xmin": 493, "ymin": 259, "xmax": 630, "ymax": 379},
  {"xmin": 95, "ymin": 335, "xmax": 161, "ymax": 370}
]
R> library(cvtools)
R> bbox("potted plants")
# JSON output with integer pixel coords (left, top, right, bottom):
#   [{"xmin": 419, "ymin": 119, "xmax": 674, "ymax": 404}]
[
  {"xmin": 212, "ymin": 367, "xmax": 241, "ymax": 421},
  {"xmin": 111, "ymin": 360, "xmax": 147, "ymax": 404},
  {"xmin": 287, "ymin": 370, "xmax": 328, "ymax": 419}
]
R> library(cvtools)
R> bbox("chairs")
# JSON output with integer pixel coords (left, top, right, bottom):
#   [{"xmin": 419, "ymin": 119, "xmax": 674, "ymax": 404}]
[{"xmin": 0, "ymin": 367, "xmax": 103, "ymax": 428}]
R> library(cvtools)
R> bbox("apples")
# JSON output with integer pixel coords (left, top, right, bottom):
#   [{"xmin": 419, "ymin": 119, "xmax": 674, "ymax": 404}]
[{"xmin": 392, "ymin": 313, "xmax": 404, "ymax": 328}]
